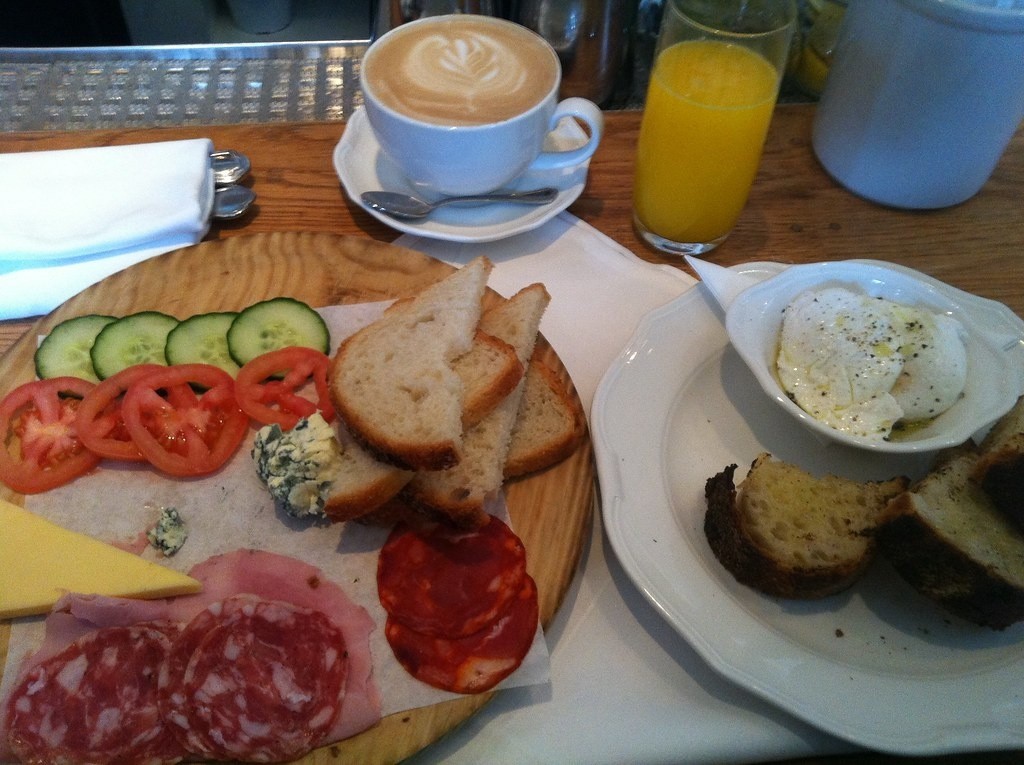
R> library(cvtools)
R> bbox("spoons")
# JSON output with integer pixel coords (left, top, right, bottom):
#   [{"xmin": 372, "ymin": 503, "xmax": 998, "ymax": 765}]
[{"xmin": 360, "ymin": 188, "xmax": 561, "ymax": 220}]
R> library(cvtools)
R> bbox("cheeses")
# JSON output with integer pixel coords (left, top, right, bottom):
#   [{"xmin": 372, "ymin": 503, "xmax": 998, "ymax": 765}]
[{"xmin": 0, "ymin": 502, "xmax": 203, "ymax": 620}]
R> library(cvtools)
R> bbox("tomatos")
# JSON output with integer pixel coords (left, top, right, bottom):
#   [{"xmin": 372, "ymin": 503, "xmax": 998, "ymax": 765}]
[{"xmin": 0, "ymin": 346, "xmax": 333, "ymax": 494}]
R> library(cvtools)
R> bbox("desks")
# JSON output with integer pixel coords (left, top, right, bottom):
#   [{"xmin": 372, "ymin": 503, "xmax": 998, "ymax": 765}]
[{"xmin": 2, "ymin": 100, "xmax": 1024, "ymax": 765}]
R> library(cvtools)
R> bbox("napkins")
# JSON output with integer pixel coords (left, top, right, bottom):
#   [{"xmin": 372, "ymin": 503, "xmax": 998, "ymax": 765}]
[{"xmin": 0, "ymin": 138, "xmax": 216, "ymax": 320}]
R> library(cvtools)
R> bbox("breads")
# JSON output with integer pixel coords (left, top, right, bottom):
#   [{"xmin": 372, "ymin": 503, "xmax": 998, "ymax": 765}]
[
  {"xmin": 280, "ymin": 254, "xmax": 583, "ymax": 521},
  {"xmin": 704, "ymin": 396, "xmax": 1024, "ymax": 630}
]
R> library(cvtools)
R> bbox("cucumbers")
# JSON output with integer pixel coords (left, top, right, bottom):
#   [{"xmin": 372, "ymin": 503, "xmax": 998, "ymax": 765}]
[{"xmin": 34, "ymin": 297, "xmax": 331, "ymax": 399}]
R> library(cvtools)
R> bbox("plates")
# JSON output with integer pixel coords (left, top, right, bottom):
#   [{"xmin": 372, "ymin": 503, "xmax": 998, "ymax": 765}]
[
  {"xmin": 725, "ymin": 260, "xmax": 1017, "ymax": 453},
  {"xmin": 1, "ymin": 231, "xmax": 588, "ymax": 764},
  {"xmin": 590, "ymin": 258, "xmax": 1024, "ymax": 757},
  {"xmin": 332, "ymin": 105, "xmax": 592, "ymax": 244}
]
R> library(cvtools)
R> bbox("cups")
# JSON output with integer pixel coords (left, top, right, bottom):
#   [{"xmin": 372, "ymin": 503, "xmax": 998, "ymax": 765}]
[
  {"xmin": 812, "ymin": 0, "xmax": 1024, "ymax": 212},
  {"xmin": 631, "ymin": 1, "xmax": 798, "ymax": 256},
  {"xmin": 361, "ymin": 13, "xmax": 605, "ymax": 203}
]
